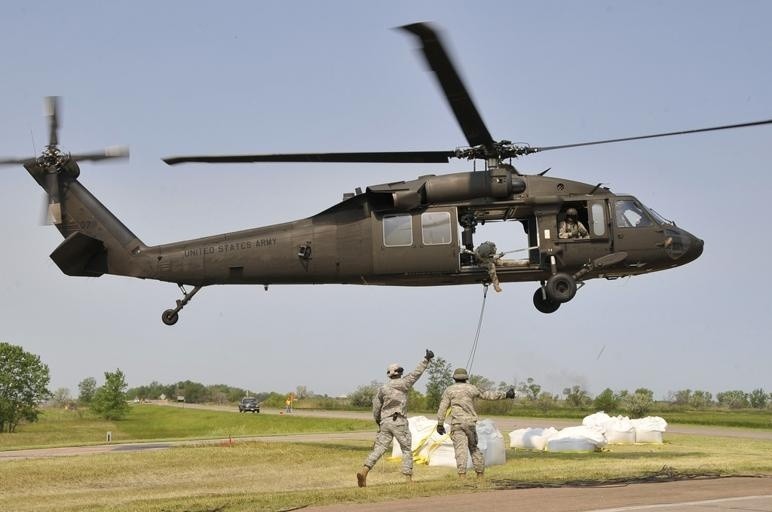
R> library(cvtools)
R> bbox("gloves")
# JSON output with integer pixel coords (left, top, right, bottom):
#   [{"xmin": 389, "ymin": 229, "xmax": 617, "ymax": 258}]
[
  {"xmin": 437, "ymin": 424, "xmax": 446, "ymax": 436},
  {"xmin": 425, "ymin": 349, "xmax": 434, "ymax": 361},
  {"xmin": 505, "ymin": 388, "xmax": 515, "ymax": 400}
]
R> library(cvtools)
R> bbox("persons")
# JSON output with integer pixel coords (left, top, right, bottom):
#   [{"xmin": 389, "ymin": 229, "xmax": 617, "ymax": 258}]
[
  {"xmin": 286, "ymin": 397, "xmax": 291, "ymax": 413},
  {"xmin": 437, "ymin": 368, "xmax": 515, "ymax": 480},
  {"xmin": 356, "ymin": 349, "xmax": 435, "ymax": 487},
  {"xmin": 474, "ymin": 241, "xmax": 503, "ymax": 293},
  {"xmin": 558, "ymin": 207, "xmax": 589, "ymax": 239}
]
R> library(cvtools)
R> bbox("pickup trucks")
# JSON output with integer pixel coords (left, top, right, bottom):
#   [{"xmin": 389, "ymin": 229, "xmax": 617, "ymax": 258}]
[{"xmin": 239, "ymin": 397, "xmax": 259, "ymax": 413}]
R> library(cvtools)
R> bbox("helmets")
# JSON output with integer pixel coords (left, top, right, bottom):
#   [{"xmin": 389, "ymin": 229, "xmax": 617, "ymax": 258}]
[
  {"xmin": 453, "ymin": 367, "xmax": 469, "ymax": 381},
  {"xmin": 387, "ymin": 364, "xmax": 404, "ymax": 378},
  {"xmin": 565, "ymin": 208, "xmax": 577, "ymax": 219},
  {"xmin": 479, "ymin": 243, "xmax": 491, "ymax": 257}
]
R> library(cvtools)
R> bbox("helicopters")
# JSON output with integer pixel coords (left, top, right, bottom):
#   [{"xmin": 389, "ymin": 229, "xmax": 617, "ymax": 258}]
[{"xmin": 0, "ymin": 22, "xmax": 772, "ymax": 326}]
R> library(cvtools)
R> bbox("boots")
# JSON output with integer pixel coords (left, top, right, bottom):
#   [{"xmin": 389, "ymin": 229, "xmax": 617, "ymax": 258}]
[{"xmin": 356, "ymin": 466, "xmax": 369, "ymax": 487}]
